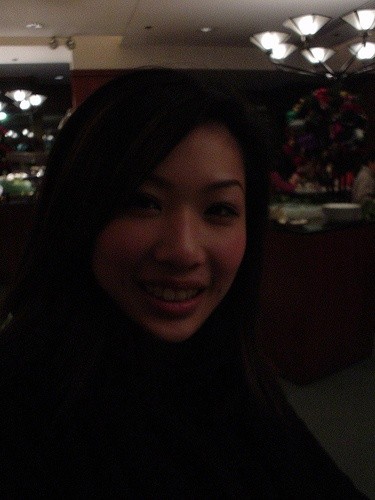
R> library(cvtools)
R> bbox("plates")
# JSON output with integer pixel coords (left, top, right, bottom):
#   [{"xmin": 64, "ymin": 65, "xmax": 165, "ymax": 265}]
[{"xmin": 278, "ymin": 219, "xmax": 306, "ymax": 228}]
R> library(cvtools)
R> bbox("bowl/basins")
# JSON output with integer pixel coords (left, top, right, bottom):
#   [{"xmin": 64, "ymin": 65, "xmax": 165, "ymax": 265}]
[
  {"xmin": 323, "ymin": 201, "xmax": 361, "ymax": 222},
  {"xmin": 1, "ymin": 173, "xmax": 41, "ymax": 194}
]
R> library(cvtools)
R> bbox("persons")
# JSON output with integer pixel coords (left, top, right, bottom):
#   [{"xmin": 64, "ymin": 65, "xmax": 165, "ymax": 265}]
[
  {"xmin": 263, "ymin": 132, "xmax": 375, "ymax": 205},
  {"xmin": 1, "ymin": 69, "xmax": 374, "ymax": 500}
]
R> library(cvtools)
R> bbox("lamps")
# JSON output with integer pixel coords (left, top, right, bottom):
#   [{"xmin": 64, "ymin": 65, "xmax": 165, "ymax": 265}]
[{"xmin": 247, "ymin": 7, "xmax": 375, "ymax": 89}]
